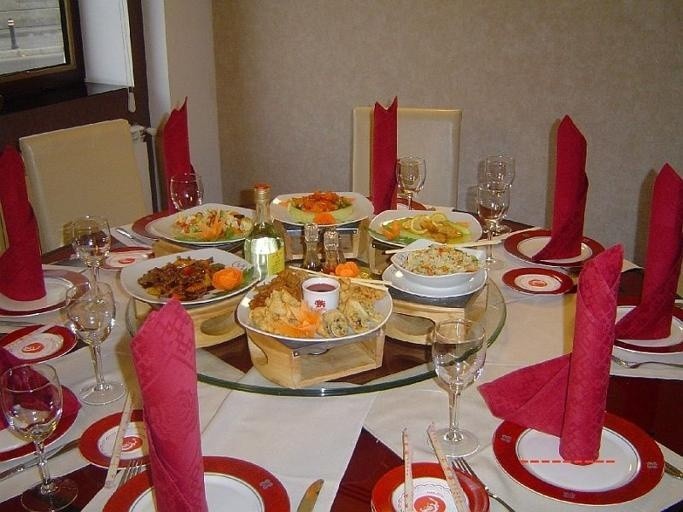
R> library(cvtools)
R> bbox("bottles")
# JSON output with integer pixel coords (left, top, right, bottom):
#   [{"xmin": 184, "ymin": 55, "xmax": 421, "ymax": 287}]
[
  {"xmin": 322, "ymin": 231, "xmax": 338, "ymax": 276},
  {"xmin": 244, "ymin": 185, "xmax": 285, "ymax": 283},
  {"xmin": 302, "ymin": 223, "xmax": 321, "ymax": 271}
]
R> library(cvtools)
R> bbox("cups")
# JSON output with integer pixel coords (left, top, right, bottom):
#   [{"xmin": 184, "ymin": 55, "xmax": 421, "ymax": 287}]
[
  {"xmin": 64, "ymin": 281, "xmax": 127, "ymax": 407},
  {"xmin": 396, "ymin": 155, "xmax": 427, "ymax": 210},
  {"xmin": 483, "ymin": 155, "xmax": 517, "ymax": 183},
  {"xmin": 300, "ymin": 277, "xmax": 340, "ymax": 311},
  {"xmin": 170, "ymin": 172, "xmax": 203, "ymax": 213},
  {"xmin": 70, "ymin": 214, "xmax": 112, "ymax": 281},
  {"xmin": 428, "ymin": 318, "xmax": 488, "ymax": 459},
  {"xmin": 0, "ymin": 361, "xmax": 80, "ymax": 512},
  {"xmin": 473, "ymin": 184, "xmax": 512, "ymax": 272}
]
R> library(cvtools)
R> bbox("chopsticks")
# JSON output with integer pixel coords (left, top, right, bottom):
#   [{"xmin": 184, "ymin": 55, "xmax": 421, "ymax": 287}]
[
  {"xmin": 384, "ymin": 240, "xmax": 502, "ymax": 254},
  {"xmin": 102, "ymin": 390, "xmax": 136, "ymax": 488},
  {"xmin": 288, "ymin": 264, "xmax": 393, "ymax": 292},
  {"xmin": 425, "ymin": 426, "xmax": 473, "ymax": 511},
  {"xmin": 2, "ymin": 312, "xmax": 69, "ymax": 352},
  {"xmin": 402, "ymin": 426, "xmax": 414, "ymax": 512}
]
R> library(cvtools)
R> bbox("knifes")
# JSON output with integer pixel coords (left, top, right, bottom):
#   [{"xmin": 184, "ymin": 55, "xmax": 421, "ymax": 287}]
[
  {"xmin": 0, "ymin": 435, "xmax": 80, "ymax": 481},
  {"xmin": 296, "ymin": 476, "xmax": 326, "ymax": 511},
  {"xmin": 115, "ymin": 227, "xmax": 150, "ymax": 245}
]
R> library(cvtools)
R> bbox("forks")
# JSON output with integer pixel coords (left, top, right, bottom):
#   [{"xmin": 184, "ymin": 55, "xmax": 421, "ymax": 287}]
[
  {"xmin": 448, "ymin": 457, "xmax": 512, "ymax": 510},
  {"xmin": 610, "ymin": 356, "xmax": 683, "ymax": 370}
]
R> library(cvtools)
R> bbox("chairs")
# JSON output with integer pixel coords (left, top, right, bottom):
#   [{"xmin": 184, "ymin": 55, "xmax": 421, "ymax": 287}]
[
  {"xmin": 19, "ymin": 119, "xmax": 154, "ymax": 257},
  {"xmin": 353, "ymin": 105, "xmax": 462, "ymax": 209}
]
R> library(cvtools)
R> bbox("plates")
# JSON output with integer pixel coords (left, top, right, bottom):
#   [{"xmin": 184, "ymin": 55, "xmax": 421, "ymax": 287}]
[
  {"xmin": 118, "ymin": 247, "xmax": 261, "ymax": 306},
  {"xmin": 367, "ymin": 210, "xmax": 484, "ymax": 248},
  {"xmin": 501, "ymin": 228, "xmax": 607, "ymax": 268},
  {"xmin": 100, "ymin": 455, "xmax": 293, "ymax": 512},
  {"xmin": 612, "ymin": 295, "xmax": 683, "ymax": 356},
  {"xmin": 371, "ymin": 461, "xmax": 490, "ymax": 512},
  {"xmin": 235, "ymin": 273, "xmax": 394, "ymax": 344},
  {"xmin": 147, "ymin": 203, "xmax": 258, "ymax": 246},
  {"xmin": 0, "ymin": 383, "xmax": 80, "ymax": 462},
  {"xmin": 268, "ymin": 191, "xmax": 376, "ymax": 228},
  {"xmin": 0, "ymin": 323, "xmax": 78, "ymax": 371},
  {"xmin": 492, "ymin": 408, "xmax": 665, "ymax": 508},
  {"xmin": 2, "ymin": 267, "xmax": 91, "ymax": 318},
  {"xmin": 398, "ymin": 197, "xmax": 426, "ymax": 209},
  {"xmin": 501, "ymin": 267, "xmax": 576, "ymax": 295},
  {"xmin": 77, "ymin": 408, "xmax": 150, "ymax": 470},
  {"xmin": 100, "ymin": 245, "xmax": 156, "ymax": 270},
  {"xmin": 380, "ymin": 265, "xmax": 486, "ymax": 298}
]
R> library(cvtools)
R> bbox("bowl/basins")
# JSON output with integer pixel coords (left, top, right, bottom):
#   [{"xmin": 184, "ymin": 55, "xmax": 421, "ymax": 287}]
[{"xmin": 389, "ymin": 236, "xmax": 488, "ymax": 291}]
